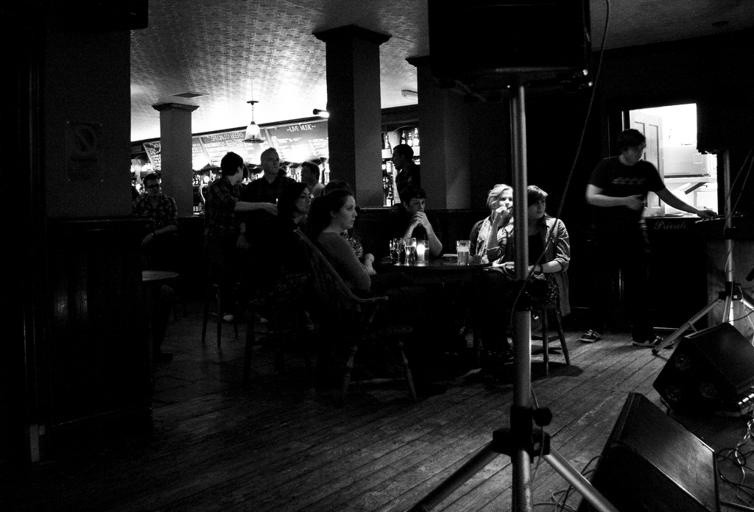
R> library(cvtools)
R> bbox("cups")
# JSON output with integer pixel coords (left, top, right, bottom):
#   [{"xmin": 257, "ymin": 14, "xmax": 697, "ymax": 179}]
[
  {"xmin": 456, "ymin": 241, "xmax": 471, "ymax": 265},
  {"xmin": 651, "ymin": 205, "xmax": 666, "ymax": 216},
  {"xmin": 417, "ymin": 240, "xmax": 430, "ymax": 262}
]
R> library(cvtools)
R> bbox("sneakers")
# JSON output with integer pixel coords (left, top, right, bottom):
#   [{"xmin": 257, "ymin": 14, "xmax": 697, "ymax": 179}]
[
  {"xmin": 577, "ymin": 328, "xmax": 601, "ymax": 343},
  {"xmin": 632, "ymin": 333, "xmax": 675, "ymax": 348}
]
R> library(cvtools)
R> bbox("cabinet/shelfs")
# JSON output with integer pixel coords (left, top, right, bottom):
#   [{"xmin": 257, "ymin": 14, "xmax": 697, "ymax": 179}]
[{"xmin": 21, "ymin": 218, "xmax": 155, "ymax": 468}]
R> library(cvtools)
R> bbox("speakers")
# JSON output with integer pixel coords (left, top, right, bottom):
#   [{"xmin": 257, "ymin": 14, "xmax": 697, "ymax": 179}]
[
  {"xmin": 579, "ymin": 389, "xmax": 724, "ymax": 511},
  {"xmin": 696, "ymin": 99, "xmax": 754, "ymax": 151},
  {"xmin": 427, "ymin": 0, "xmax": 592, "ymax": 93},
  {"xmin": 654, "ymin": 290, "xmax": 754, "ymax": 412}
]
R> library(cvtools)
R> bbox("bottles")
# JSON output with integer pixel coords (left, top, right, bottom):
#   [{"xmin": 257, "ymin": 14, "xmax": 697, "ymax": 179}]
[{"xmin": 386, "ymin": 185, "xmax": 395, "ymax": 207}]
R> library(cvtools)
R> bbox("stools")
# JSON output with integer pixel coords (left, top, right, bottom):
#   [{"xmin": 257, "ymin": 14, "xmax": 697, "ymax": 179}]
[
  {"xmin": 200, "ymin": 281, "xmax": 239, "ymax": 349},
  {"xmin": 244, "ymin": 301, "xmax": 306, "ymax": 382}
]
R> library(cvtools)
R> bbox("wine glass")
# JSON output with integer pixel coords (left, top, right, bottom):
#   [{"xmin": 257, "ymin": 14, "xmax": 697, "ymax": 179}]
[{"xmin": 389, "ymin": 238, "xmax": 416, "ymax": 266}]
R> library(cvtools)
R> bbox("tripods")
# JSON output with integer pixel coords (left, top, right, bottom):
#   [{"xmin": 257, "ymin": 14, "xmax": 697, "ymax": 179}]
[
  {"xmin": 652, "ymin": 150, "xmax": 754, "ymax": 357},
  {"xmin": 408, "ymin": 93, "xmax": 618, "ymax": 512}
]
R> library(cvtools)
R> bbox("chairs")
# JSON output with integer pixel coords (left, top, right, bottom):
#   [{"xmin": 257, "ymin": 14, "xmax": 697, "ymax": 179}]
[
  {"xmin": 293, "ymin": 228, "xmax": 418, "ymax": 407},
  {"xmin": 530, "ymin": 302, "xmax": 571, "ymax": 380}
]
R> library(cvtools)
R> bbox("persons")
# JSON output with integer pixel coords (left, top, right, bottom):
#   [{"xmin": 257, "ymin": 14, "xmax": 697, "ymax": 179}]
[
  {"xmin": 576, "ymin": 128, "xmax": 718, "ymax": 349},
  {"xmin": 132, "ymin": 143, "xmax": 574, "ymax": 407}
]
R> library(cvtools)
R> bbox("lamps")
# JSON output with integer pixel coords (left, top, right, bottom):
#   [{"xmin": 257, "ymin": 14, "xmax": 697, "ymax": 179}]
[{"xmin": 242, "ymin": 100, "xmax": 264, "ymax": 144}]
